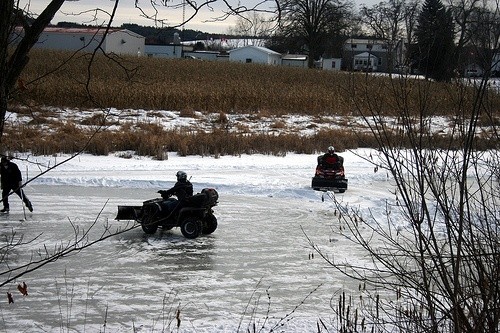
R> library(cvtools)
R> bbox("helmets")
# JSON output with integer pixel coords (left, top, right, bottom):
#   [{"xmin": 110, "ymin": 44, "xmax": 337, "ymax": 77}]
[
  {"xmin": 176, "ymin": 171, "xmax": 187, "ymax": 180},
  {"xmin": 1, "ymin": 156, "xmax": 10, "ymax": 165},
  {"xmin": 328, "ymin": 145, "xmax": 335, "ymax": 155}
]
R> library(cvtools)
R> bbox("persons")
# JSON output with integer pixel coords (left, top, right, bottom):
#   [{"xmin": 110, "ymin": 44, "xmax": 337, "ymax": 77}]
[
  {"xmin": 316, "ymin": 146, "xmax": 344, "ymax": 177},
  {"xmin": 0, "ymin": 156, "xmax": 33, "ymax": 214},
  {"xmin": 158, "ymin": 170, "xmax": 193, "ymax": 224}
]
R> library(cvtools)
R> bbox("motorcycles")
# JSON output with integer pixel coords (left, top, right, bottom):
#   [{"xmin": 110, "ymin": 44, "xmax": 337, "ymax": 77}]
[
  {"xmin": 114, "ymin": 188, "xmax": 219, "ymax": 239},
  {"xmin": 312, "ymin": 154, "xmax": 348, "ymax": 193}
]
